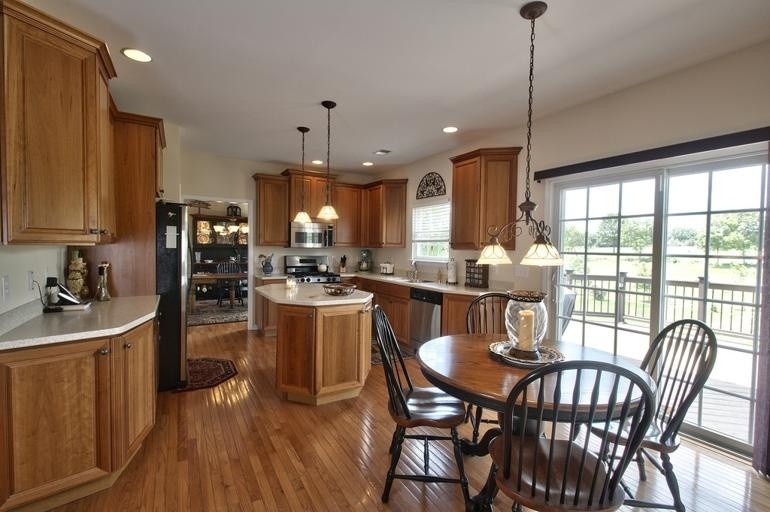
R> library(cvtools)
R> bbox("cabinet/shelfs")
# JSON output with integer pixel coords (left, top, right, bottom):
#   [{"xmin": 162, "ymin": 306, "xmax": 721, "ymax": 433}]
[
  {"xmin": 156, "ymin": 201, "xmax": 189, "ymax": 391},
  {"xmin": 448, "ymin": 145, "xmax": 523, "ymax": 250},
  {"xmin": 68, "ymin": 113, "xmax": 168, "ymax": 296},
  {"xmin": 1, "ymin": 312, "xmax": 157, "ymax": 512},
  {"xmin": 190, "ymin": 213, "xmax": 248, "ymax": 301},
  {"xmin": 255, "ymin": 272, "xmax": 510, "ymax": 356},
  {"xmin": 256, "ymin": 279, "xmax": 374, "ymax": 405},
  {"xmin": 0, "ymin": 0, "xmax": 119, "ymax": 245},
  {"xmin": 252, "ymin": 169, "xmax": 408, "ymax": 248}
]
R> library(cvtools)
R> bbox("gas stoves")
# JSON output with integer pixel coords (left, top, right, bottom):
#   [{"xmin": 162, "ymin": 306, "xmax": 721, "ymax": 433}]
[{"xmin": 285, "ymin": 272, "xmax": 340, "ymax": 284}]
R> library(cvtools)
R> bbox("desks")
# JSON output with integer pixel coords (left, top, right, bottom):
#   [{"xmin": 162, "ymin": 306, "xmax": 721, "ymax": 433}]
[
  {"xmin": 416, "ymin": 334, "xmax": 652, "ymax": 511},
  {"xmin": 189, "ymin": 273, "xmax": 249, "ymax": 315}
]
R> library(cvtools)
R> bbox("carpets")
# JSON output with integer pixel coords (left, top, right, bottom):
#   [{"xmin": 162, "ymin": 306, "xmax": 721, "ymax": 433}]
[
  {"xmin": 186, "ymin": 298, "xmax": 248, "ymax": 326},
  {"xmin": 173, "ymin": 357, "xmax": 238, "ymax": 393}
]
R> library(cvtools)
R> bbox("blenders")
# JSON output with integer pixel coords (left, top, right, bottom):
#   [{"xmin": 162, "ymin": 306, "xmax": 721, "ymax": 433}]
[{"xmin": 359, "ymin": 250, "xmax": 371, "ymax": 271}]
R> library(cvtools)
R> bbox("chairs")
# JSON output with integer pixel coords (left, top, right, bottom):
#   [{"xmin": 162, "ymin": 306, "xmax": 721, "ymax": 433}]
[
  {"xmin": 571, "ymin": 320, "xmax": 716, "ymax": 512},
  {"xmin": 372, "ymin": 305, "xmax": 471, "ymax": 508},
  {"xmin": 465, "ymin": 291, "xmax": 509, "ymax": 442},
  {"xmin": 490, "ymin": 362, "xmax": 656, "ymax": 511},
  {"xmin": 216, "ymin": 260, "xmax": 244, "ymax": 308}
]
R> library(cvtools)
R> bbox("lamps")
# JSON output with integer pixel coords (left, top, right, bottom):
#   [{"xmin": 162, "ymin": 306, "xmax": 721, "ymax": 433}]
[
  {"xmin": 475, "ymin": 2, "xmax": 565, "ymax": 267},
  {"xmin": 292, "ymin": 126, "xmax": 312, "ymax": 223},
  {"xmin": 317, "ymin": 100, "xmax": 339, "ymax": 221},
  {"xmin": 212, "ymin": 217, "xmax": 249, "ymax": 236}
]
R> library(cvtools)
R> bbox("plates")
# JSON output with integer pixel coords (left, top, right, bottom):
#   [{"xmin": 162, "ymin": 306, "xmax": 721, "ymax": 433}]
[{"xmin": 204, "ymin": 260, "xmax": 214, "ymax": 263}]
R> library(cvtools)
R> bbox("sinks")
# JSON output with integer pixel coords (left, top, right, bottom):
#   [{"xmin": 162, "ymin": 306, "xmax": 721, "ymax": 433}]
[{"xmin": 404, "ymin": 279, "xmax": 435, "ymax": 283}]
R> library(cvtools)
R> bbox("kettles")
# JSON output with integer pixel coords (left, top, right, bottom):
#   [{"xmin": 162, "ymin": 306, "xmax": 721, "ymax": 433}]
[{"xmin": 318, "ymin": 262, "xmax": 327, "ymax": 272}]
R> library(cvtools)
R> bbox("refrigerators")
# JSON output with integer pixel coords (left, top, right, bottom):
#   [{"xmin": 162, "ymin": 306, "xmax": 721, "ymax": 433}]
[{"xmin": 155, "ymin": 201, "xmax": 194, "ymax": 391}]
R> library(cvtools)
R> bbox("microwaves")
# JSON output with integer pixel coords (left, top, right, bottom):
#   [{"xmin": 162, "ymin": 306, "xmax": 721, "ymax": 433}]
[{"xmin": 290, "ymin": 222, "xmax": 334, "ymax": 248}]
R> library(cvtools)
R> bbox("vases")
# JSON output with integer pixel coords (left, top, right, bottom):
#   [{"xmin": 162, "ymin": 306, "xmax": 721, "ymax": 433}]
[{"xmin": 259, "ymin": 253, "xmax": 273, "ymax": 276}]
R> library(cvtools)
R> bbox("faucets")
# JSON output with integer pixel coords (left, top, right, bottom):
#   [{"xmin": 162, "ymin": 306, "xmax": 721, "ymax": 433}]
[{"xmin": 409, "ymin": 261, "xmax": 420, "ymax": 280}]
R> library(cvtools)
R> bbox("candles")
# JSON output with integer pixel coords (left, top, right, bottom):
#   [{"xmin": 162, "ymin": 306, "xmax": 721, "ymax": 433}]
[{"xmin": 517, "ymin": 308, "xmax": 536, "ymax": 347}]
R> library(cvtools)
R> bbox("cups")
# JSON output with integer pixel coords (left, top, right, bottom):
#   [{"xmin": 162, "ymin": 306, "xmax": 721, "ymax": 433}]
[{"xmin": 230, "ymin": 257, "xmax": 236, "ymax": 263}]
